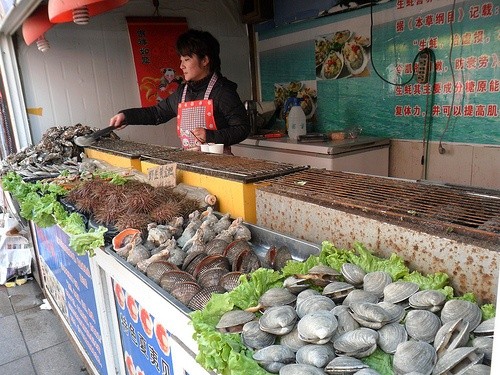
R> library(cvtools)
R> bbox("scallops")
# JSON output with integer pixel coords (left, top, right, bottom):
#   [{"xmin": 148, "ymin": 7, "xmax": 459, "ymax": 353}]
[{"xmin": 146, "ymin": 237, "xmax": 292, "ymax": 312}]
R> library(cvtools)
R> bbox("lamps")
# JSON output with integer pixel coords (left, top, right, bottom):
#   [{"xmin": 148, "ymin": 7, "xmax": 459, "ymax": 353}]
[
  {"xmin": 48, "ymin": 0, "xmax": 128, "ymax": 26},
  {"xmin": 22, "ymin": 12, "xmax": 55, "ymax": 53}
]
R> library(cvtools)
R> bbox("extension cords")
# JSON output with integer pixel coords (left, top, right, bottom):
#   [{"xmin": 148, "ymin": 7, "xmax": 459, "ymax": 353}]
[{"xmin": 417, "ymin": 52, "xmax": 429, "ymax": 83}]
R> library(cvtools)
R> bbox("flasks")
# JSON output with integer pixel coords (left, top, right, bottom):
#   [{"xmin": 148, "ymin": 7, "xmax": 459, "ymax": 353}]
[{"xmin": 243, "ymin": 100, "xmax": 257, "ymax": 138}]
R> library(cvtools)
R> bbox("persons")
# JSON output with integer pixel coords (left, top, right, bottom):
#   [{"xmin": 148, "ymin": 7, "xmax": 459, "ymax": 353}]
[{"xmin": 110, "ymin": 29, "xmax": 251, "ymax": 156}]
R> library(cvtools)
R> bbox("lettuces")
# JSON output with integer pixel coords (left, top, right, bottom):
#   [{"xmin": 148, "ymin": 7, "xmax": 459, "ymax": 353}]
[
  {"xmin": 189, "ymin": 242, "xmax": 496, "ymax": 375},
  {"xmin": 1, "ymin": 167, "xmax": 128, "ymax": 256}
]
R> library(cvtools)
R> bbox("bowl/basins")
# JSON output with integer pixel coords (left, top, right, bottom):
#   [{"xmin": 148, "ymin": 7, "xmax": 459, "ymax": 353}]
[{"xmin": 345, "ymin": 127, "xmax": 362, "ymax": 139}]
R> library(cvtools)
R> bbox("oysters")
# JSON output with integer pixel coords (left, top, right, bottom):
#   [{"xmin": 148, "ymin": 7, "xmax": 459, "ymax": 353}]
[{"xmin": 0, "ymin": 123, "xmax": 121, "ymax": 167}]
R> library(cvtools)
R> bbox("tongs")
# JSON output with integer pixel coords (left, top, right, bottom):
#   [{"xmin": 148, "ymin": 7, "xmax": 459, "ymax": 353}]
[{"xmin": 83, "ymin": 120, "xmax": 129, "ymax": 142}]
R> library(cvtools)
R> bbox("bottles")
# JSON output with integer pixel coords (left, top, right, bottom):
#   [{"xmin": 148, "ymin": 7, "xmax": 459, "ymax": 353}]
[{"xmin": 173, "ymin": 183, "xmax": 217, "ymax": 208}]
